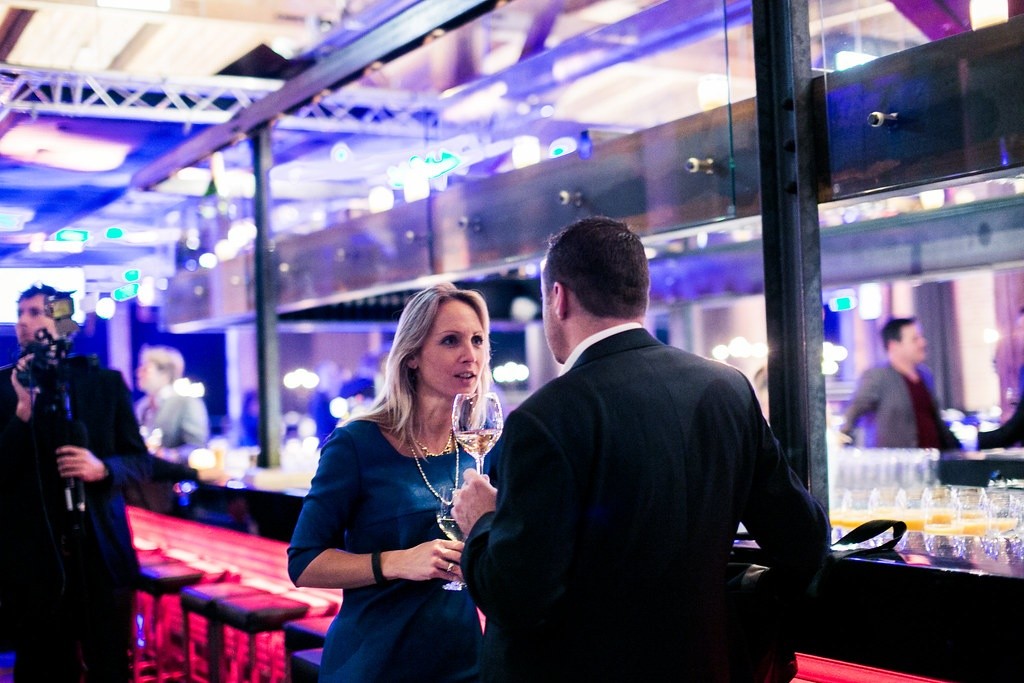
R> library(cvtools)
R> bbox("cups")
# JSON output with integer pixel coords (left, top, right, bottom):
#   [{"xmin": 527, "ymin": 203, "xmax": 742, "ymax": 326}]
[{"xmin": 829, "ymin": 483, "xmax": 1024, "ymax": 565}]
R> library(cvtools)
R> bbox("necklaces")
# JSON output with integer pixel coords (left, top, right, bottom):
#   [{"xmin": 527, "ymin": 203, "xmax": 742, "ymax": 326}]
[{"xmin": 406, "ymin": 430, "xmax": 460, "ymax": 505}]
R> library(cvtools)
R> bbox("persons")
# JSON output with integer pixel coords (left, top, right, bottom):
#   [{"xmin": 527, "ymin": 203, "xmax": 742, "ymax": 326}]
[
  {"xmin": 450, "ymin": 219, "xmax": 831, "ymax": 683},
  {"xmin": 0, "ymin": 286, "xmax": 148, "ymax": 681},
  {"xmin": 288, "ymin": 284, "xmax": 503, "ymax": 683},
  {"xmin": 841, "ymin": 318, "xmax": 965, "ymax": 449},
  {"xmin": 135, "ymin": 347, "xmax": 209, "ymax": 463},
  {"xmin": 976, "ymin": 308, "xmax": 1024, "ymax": 451}
]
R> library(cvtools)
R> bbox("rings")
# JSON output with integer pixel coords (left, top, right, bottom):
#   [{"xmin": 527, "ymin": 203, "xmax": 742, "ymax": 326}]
[{"xmin": 448, "ymin": 563, "xmax": 454, "ymax": 572}]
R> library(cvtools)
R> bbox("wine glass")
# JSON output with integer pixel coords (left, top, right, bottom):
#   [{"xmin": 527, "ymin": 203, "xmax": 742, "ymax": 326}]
[
  {"xmin": 451, "ymin": 391, "xmax": 504, "ymax": 477},
  {"xmin": 437, "ymin": 484, "xmax": 470, "ymax": 590}
]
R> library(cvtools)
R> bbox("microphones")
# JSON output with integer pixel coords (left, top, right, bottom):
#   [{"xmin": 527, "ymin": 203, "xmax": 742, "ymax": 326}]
[{"xmin": 62, "ymin": 420, "xmax": 86, "ymax": 514}]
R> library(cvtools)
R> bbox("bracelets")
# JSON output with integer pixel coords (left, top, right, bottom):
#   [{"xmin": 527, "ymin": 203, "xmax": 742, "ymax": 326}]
[{"xmin": 372, "ymin": 550, "xmax": 387, "ymax": 584}]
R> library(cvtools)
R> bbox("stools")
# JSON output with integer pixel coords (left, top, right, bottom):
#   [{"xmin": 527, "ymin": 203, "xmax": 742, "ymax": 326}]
[{"xmin": 133, "ymin": 534, "xmax": 338, "ymax": 683}]
